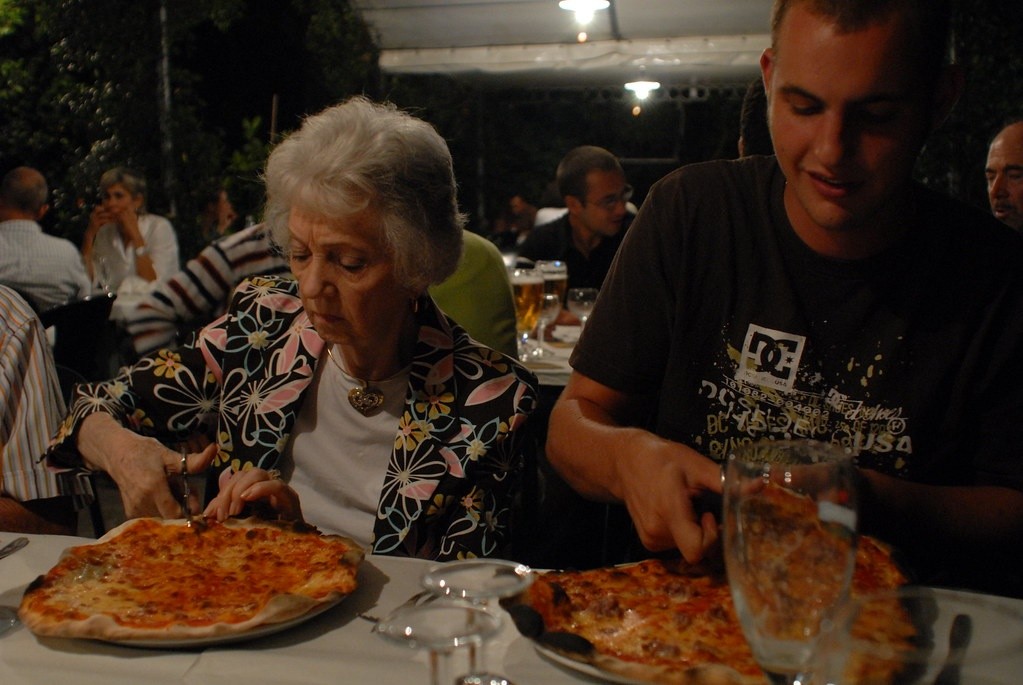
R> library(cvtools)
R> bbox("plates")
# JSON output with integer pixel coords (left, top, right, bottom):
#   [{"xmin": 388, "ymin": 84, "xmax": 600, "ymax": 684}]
[
  {"xmin": 530, "ymin": 639, "xmax": 639, "ymax": 685},
  {"xmin": 110, "ymin": 592, "xmax": 347, "ymax": 645}
]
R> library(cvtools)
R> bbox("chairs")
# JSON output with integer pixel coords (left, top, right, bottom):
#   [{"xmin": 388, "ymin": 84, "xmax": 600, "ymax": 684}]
[{"xmin": 36, "ymin": 295, "xmax": 119, "ymax": 402}]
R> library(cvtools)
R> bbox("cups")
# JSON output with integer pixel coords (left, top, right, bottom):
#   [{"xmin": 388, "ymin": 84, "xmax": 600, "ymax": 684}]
[{"xmin": 829, "ymin": 589, "xmax": 1023, "ymax": 685}]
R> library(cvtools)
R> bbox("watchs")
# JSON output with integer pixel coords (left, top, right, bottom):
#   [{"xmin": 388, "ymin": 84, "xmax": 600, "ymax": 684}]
[{"xmin": 134, "ymin": 245, "xmax": 146, "ymax": 257}]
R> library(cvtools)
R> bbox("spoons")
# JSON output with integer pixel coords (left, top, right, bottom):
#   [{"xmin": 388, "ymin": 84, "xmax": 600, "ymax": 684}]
[{"xmin": 0, "ymin": 604, "xmax": 31, "ymax": 637}]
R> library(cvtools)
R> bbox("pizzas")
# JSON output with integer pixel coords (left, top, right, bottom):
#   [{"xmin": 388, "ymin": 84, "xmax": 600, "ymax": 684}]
[
  {"xmin": 533, "ymin": 477, "xmax": 922, "ymax": 685},
  {"xmin": 17, "ymin": 513, "xmax": 366, "ymax": 640}
]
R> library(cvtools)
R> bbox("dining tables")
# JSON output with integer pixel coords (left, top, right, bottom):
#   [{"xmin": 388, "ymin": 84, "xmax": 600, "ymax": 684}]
[
  {"xmin": 516, "ymin": 311, "xmax": 592, "ymax": 387},
  {"xmin": 0, "ymin": 530, "xmax": 1023, "ymax": 685}
]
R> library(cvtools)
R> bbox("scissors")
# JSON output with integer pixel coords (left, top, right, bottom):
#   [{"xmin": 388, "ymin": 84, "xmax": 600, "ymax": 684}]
[{"xmin": 180, "ymin": 447, "xmax": 196, "ymax": 525}]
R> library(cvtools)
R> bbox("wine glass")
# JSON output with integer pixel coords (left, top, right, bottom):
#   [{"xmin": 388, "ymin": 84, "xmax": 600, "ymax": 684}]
[
  {"xmin": 505, "ymin": 267, "xmax": 544, "ymax": 362},
  {"xmin": 567, "ymin": 287, "xmax": 599, "ymax": 333},
  {"xmin": 376, "ymin": 600, "xmax": 507, "ymax": 685},
  {"xmin": 535, "ymin": 260, "xmax": 568, "ymax": 309},
  {"xmin": 531, "ymin": 293, "xmax": 559, "ymax": 359},
  {"xmin": 418, "ymin": 561, "xmax": 535, "ymax": 685},
  {"xmin": 723, "ymin": 441, "xmax": 860, "ymax": 684}
]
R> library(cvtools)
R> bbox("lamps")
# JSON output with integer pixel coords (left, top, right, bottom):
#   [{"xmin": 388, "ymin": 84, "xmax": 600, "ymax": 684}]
[
  {"xmin": 625, "ymin": 67, "xmax": 662, "ymax": 101},
  {"xmin": 558, "ymin": 0, "xmax": 611, "ymax": 26}
]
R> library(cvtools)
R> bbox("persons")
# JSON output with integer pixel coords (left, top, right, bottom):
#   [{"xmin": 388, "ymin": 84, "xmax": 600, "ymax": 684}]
[
  {"xmin": 0, "ymin": 146, "xmax": 637, "ymax": 364},
  {"xmin": 34, "ymin": 94, "xmax": 553, "ymax": 563},
  {"xmin": 737, "ymin": 74, "xmax": 775, "ymax": 157},
  {"xmin": 546, "ymin": 0, "xmax": 1023, "ymax": 599},
  {"xmin": 0, "ymin": 285, "xmax": 93, "ymax": 536},
  {"xmin": 986, "ymin": 120, "xmax": 1023, "ymax": 235}
]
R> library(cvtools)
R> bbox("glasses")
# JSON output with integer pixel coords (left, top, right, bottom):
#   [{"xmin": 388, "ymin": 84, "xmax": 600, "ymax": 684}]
[{"xmin": 586, "ymin": 184, "xmax": 634, "ymax": 209}]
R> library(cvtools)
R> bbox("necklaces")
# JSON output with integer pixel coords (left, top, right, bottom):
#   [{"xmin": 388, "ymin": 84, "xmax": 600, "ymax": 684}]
[{"xmin": 324, "ymin": 343, "xmax": 411, "ymax": 417}]
